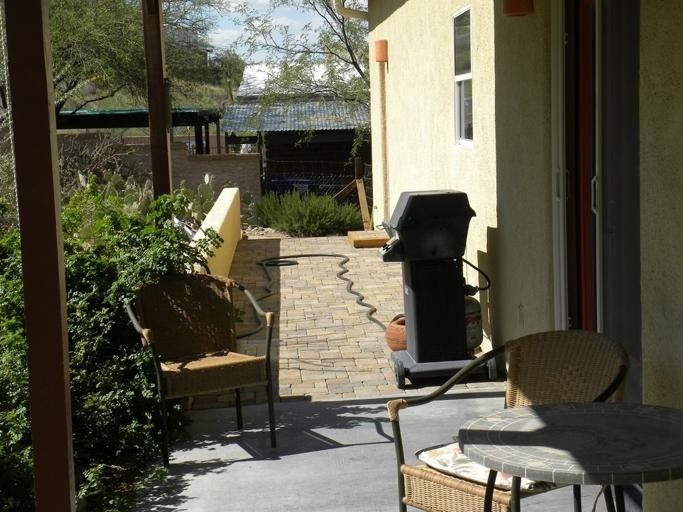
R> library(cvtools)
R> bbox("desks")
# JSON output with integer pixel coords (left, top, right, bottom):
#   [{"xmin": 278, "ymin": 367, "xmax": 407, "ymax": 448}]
[{"xmin": 459, "ymin": 401, "xmax": 683, "ymax": 512}]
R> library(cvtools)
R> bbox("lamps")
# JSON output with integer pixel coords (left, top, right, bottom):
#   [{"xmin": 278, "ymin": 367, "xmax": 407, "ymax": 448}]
[
  {"xmin": 376, "ymin": 40, "xmax": 388, "ymax": 62},
  {"xmin": 503, "ymin": 0, "xmax": 534, "ymax": 17}
]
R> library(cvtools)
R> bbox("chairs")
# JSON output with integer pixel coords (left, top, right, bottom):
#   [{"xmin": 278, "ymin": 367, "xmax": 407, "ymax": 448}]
[
  {"xmin": 126, "ymin": 273, "xmax": 276, "ymax": 467},
  {"xmin": 387, "ymin": 329, "xmax": 629, "ymax": 512}
]
